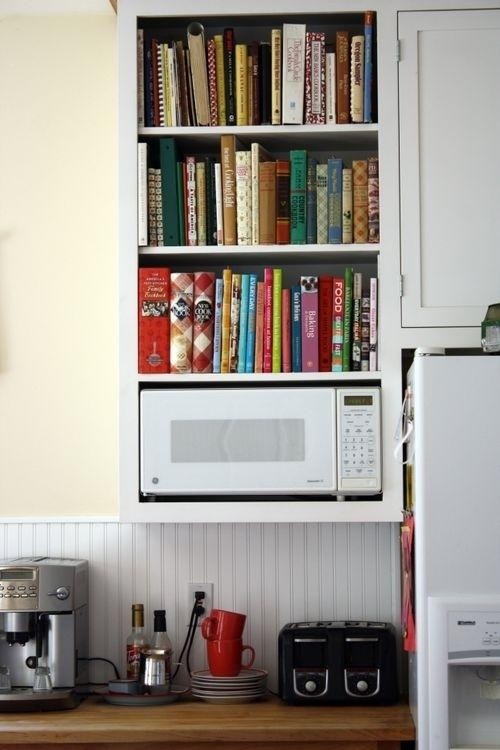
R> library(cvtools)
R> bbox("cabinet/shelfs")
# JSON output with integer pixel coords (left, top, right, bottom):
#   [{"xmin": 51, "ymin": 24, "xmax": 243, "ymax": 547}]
[
  {"xmin": 398, "ymin": 0, "xmax": 500, "ymax": 348},
  {"xmin": 116, "ymin": 3, "xmax": 404, "ymax": 522}
]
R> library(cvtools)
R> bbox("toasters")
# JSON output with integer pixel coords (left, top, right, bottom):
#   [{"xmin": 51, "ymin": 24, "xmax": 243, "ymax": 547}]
[{"xmin": 276, "ymin": 620, "xmax": 401, "ymax": 707}]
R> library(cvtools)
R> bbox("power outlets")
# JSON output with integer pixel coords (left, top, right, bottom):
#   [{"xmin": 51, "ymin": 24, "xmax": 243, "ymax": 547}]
[{"xmin": 186, "ymin": 583, "xmax": 212, "ymax": 626}]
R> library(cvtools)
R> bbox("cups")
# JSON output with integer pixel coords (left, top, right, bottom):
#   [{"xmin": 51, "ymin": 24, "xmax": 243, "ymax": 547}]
[
  {"xmin": 110, "ymin": 680, "xmax": 138, "ymax": 696},
  {"xmin": 201, "ymin": 608, "xmax": 256, "ymax": 676},
  {"xmin": 139, "ymin": 648, "xmax": 175, "ymax": 686}
]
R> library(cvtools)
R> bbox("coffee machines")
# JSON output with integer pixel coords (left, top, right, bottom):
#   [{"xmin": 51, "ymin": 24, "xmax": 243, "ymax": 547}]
[{"xmin": 0, "ymin": 558, "xmax": 89, "ymax": 711}]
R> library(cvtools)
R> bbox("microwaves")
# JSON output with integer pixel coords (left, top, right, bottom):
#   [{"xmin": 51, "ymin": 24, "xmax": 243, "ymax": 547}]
[{"xmin": 140, "ymin": 387, "xmax": 381, "ymax": 499}]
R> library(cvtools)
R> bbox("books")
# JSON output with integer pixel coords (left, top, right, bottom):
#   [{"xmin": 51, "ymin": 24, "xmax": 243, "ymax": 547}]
[
  {"xmin": 138, "ymin": 11, "xmax": 372, "ymax": 126},
  {"xmin": 137, "ymin": 135, "xmax": 379, "ymax": 247},
  {"xmin": 137, "ymin": 267, "xmax": 377, "ymax": 373}
]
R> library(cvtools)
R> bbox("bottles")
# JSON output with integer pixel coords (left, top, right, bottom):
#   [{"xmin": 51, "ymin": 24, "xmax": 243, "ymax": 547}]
[
  {"xmin": 124, "ymin": 602, "xmax": 172, "ymax": 681},
  {"xmin": 34, "ymin": 654, "xmax": 54, "ymax": 695}
]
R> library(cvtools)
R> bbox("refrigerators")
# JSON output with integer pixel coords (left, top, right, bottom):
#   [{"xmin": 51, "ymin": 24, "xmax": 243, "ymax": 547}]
[{"xmin": 404, "ymin": 355, "xmax": 499, "ymax": 750}]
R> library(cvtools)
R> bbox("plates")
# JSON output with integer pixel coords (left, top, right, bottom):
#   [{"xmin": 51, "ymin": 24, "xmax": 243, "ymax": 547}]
[{"xmin": 189, "ymin": 669, "xmax": 268, "ymax": 705}]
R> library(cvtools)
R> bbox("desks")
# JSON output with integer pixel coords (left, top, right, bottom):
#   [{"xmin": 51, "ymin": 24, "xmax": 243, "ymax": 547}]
[{"xmin": 0, "ymin": 694, "xmax": 415, "ymax": 750}]
